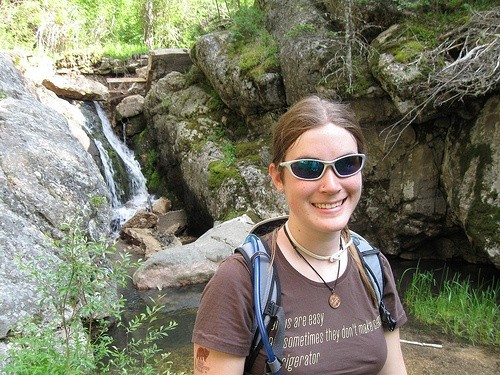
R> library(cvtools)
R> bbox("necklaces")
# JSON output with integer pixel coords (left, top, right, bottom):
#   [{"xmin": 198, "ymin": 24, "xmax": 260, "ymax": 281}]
[
  {"xmin": 282, "ymin": 220, "xmax": 343, "ymax": 309},
  {"xmin": 285, "ymin": 222, "xmax": 347, "ymax": 263}
]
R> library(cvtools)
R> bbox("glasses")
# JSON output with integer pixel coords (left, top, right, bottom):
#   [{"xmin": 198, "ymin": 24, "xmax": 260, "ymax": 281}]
[{"xmin": 278, "ymin": 154, "xmax": 367, "ymax": 181}]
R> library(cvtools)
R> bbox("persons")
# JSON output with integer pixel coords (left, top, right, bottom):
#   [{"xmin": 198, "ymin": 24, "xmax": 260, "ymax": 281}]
[{"xmin": 191, "ymin": 93, "xmax": 411, "ymax": 375}]
